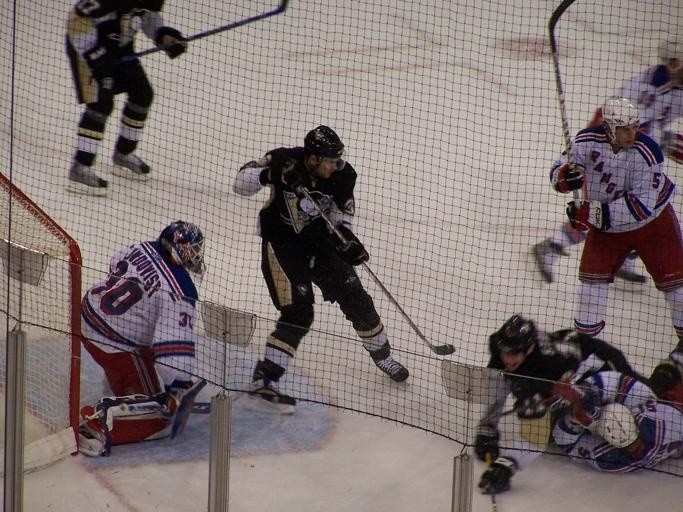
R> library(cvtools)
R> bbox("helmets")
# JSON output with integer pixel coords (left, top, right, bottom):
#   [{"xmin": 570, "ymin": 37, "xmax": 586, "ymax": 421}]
[
  {"xmin": 303, "ymin": 124, "xmax": 345, "ymax": 158},
  {"xmin": 656, "ymin": 38, "xmax": 683, "ymax": 62},
  {"xmin": 602, "ymin": 95, "xmax": 640, "ymax": 131},
  {"xmin": 494, "ymin": 312, "xmax": 539, "ymax": 352},
  {"xmin": 596, "ymin": 401, "xmax": 641, "ymax": 449},
  {"xmin": 158, "ymin": 218, "xmax": 204, "ymax": 252}
]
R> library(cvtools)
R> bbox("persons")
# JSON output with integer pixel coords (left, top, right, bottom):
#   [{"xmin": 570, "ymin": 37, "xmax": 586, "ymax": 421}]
[
  {"xmin": 232, "ymin": 123, "xmax": 409, "ymax": 409},
  {"xmin": 531, "ymin": 25, "xmax": 681, "ymax": 287},
  {"xmin": 76, "ymin": 219, "xmax": 206, "ymax": 459},
  {"xmin": 552, "ymin": 370, "xmax": 682, "ymax": 476},
  {"xmin": 63, "ymin": 0, "xmax": 189, "ymax": 190},
  {"xmin": 474, "ymin": 315, "xmax": 682, "ymax": 496},
  {"xmin": 549, "ymin": 95, "xmax": 681, "ymax": 363}
]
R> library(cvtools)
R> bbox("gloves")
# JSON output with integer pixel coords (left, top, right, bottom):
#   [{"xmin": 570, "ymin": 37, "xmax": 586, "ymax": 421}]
[
  {"xmin": 81, "ymin": 43, "xmax": 123, "ymax": 90},
  {"xmin": 267, "ymin": 158, "xmax": 312, "ymax": 193},
  {"xmin": 153, "ymin": 27, "xmax": 187, "ymax": 59},
  {"xmin": 571, "ymin": 401, "xmax": 602, "ymax": 426},
  {"xmin": 476, "ymin": 456, "xmax": 520, "ymax": 495},
  {"xmin": 565, "ymin": 198, "xmax": 610, "ymax": 232},
  {"xmin": 473, "ymin": 430, "xmax": 500, "ymax": 460},
  {"xmin": 660, "ymin": 127, "xmax": 682, "ymax": 167},
  {"xmin": 335, "ymin": 232, "xmax": 371, "ymax": 268},
  {"xmin": 549, "ymin": 161, "xmax": 586, "ymax": 195}
]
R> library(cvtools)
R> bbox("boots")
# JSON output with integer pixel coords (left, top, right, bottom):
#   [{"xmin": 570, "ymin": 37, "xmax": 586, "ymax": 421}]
[
  {"xmin": 532, "ymin": 239, "xmax": 572, "ymax": 284},
  {"xmin": 609, "ymin": 252, "xmax": 654, "ymax": 283},
  {"xmin": 55, "ymin": 420, "xmax": 110, "ymax": 459},
  {"xmin": 111, "ymin": 152, "xmax": 151, "ymax": 175},
  {"xmin": 367, "ymin": 349, "xmax": 411, "ymax": 382},
  {"xmin": 65, "ymin": 162, "xmax": 109, "ymax": 188},
  {"xmin": 244, "ymin": 360, "xmax": 298, "ymax": 407}
]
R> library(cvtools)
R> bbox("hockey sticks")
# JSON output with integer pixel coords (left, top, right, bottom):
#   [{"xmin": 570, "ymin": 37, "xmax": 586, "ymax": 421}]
[
  {"xmin": 299, "ymin": 185, "xmax": 455, "ymax": 355},
  {"xmin": 547, "ymin": 0, "xmax": 575, "ymax": 203},
  {"xmin": 115, "ymin": 0, "xmax": 286, "ymax": 64}
]
